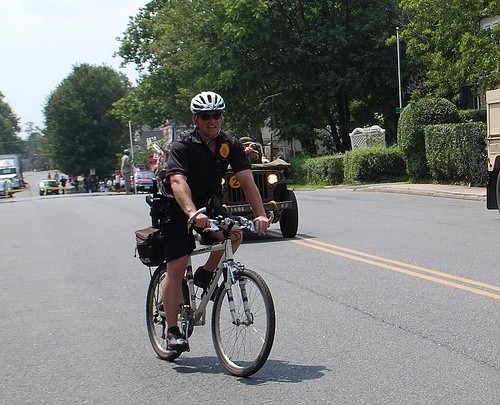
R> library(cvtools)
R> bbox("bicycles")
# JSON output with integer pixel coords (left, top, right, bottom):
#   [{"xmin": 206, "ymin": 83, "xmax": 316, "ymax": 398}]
[{"xmin": 145, "ymin": 206, "xmax": 276, "ymax": 378}]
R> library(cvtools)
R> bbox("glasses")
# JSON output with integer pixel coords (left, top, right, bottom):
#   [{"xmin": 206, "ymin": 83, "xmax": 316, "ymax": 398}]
[{"xmin": 200, "ymin": 112, "xmax": 222, "ymax": 120}]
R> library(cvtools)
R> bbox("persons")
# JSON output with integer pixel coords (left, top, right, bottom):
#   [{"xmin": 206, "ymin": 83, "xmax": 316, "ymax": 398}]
[
  {"xmin": 121, "ymin": 149, "xmax": 134, "ymax": 195},
  {"xmin": 60, "ymin": 176, "xmax": 67, "ymax": 194},
  {"xmin": 161, "ymin": 91, "xmax": 269, "ymax": 352},
  {"xmin": 48, "ymin": 173, "xmax": 51, "ymax": 179},
  {"xmin": 54, "ymin": 172, "xmax": 59, "ymax": 181},
  {"xmin": 239, "ymin": 137, "xmax": 262, "ymax": 163}
]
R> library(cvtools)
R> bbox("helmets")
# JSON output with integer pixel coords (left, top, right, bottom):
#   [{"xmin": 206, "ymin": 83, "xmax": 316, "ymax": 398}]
[{"xmin": 190, "ymin": 91, "xmax": 225, "ymax": 114}]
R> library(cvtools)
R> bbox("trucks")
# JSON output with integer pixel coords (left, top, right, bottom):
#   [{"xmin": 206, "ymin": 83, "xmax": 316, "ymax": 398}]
[
  {"xmin": 0, "ymin": 153, "xmax": 24, "ymax": 199},
  {"xmin": 485, "ymin": 88, "xmax": 500, "ymax": 212}
]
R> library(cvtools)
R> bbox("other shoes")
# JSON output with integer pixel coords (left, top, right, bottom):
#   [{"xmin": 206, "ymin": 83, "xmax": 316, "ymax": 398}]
[
  {"xmin": 164, "ymin": 326, "xmax": 189, "ymax": 351},
  {"xmin": 126, "ymin": 191, "xmax": 133, "ymax": 194},
  {"xmin": 132, "ymin": 191, "xmax": 134, "ymax": 194},
  {"xmin": 192, "ymin": 266, "xmax": 218, "ymax": 302}
]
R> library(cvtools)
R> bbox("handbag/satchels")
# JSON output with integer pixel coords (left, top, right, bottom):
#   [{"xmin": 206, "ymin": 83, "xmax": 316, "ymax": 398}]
[{"xmin": 135, "ymin": 226, "xmax": 166, "ymax": 266}]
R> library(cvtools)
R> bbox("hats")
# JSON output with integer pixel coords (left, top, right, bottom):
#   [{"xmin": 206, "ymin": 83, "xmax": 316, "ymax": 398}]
[
  {"xmin": 124, "ymin": 149, "xmax": 130, "ymax": 153},
  {"xmin": 239, "ymin": 136, "xmax": 253, "ymax": 144}
]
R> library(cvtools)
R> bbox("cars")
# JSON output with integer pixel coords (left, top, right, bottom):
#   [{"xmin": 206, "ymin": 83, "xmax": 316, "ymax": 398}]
[
  {"xmin": 38, "ymin": 179, "xmax": 60, "ymax": 196},
  {"xmin": 130, "ymin": 171, "xmax": 160, "ymax": 194},
  {"xmin": 220, "ymin": 142, "xmax": 299, "ymax": 238}
]
